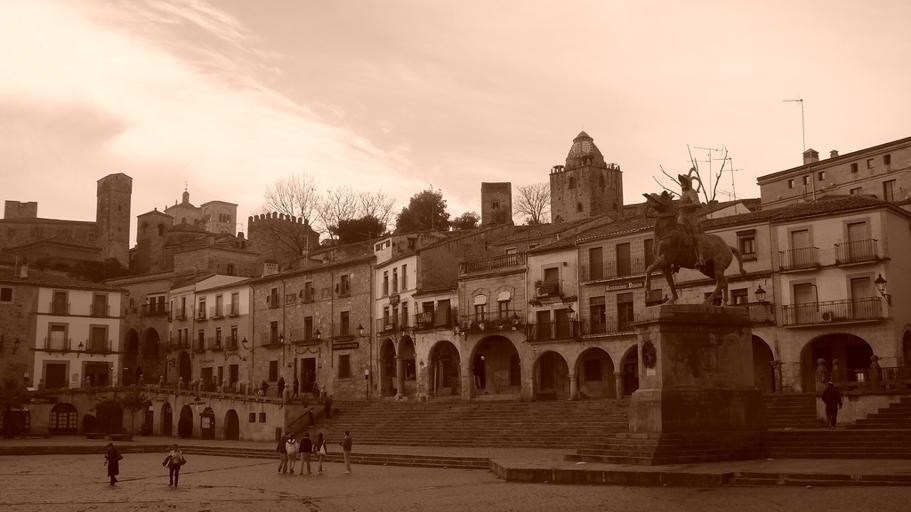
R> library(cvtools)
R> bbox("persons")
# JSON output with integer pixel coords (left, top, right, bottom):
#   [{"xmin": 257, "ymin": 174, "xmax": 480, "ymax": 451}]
[
  {"xmin": 315, "ymin": 434, "xmax": 327, "ymax": 475},
  {"xmin": 254, "ymin": 377, "xmax": 333, "ymax": 426},
  {"xmin": 676, "ymin": 174, "xmax": 706, "ymax": 267},
  {"xmin": 162, "ymin": 444, "xmax": 183, "ymax": 487},
  {"xmin": 342, "ymin": 431, "xmax": 352, "ymax": 473},
  {"xmin": 276, "ymin": 432, "xmax": 292, "ymax": 473},
  {"xmin": 104, "ymin": 443, "xmax": 122, "ymax": 485},
  {"xmin": 299, "ymin": 432, "xmax": 313, "ymax": 475},
  {"xmin": 285, "ymin": 434, "xmax": 299, "ymax": 473},
  {"xmin": 822, "ymin": 381, "xmax": 842, "ymax": 429}
]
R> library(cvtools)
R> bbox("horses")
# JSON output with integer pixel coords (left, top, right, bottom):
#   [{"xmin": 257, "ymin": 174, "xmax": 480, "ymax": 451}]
[{"xmin": 645, "ymin": 188, "xmax": 754, "ymax": 306}]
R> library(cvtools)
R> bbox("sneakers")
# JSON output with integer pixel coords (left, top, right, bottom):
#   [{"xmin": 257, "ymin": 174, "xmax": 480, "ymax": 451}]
[
  {"xmin": 167, "ymin": 483, "xmax": 177, "ymax": 487},
  {"xmin": 344, "ymin": 470, "xmax": 351, "ymax": 474}
]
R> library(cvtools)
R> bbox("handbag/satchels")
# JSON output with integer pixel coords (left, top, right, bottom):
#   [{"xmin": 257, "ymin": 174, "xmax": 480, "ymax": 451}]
[
  {"xmin": 177, "ymin": 458, "xmax": 186, "ymax": 465},
  {"xmin": 115, "ymin": 455, "xmax": 123, "ymax": 460},
  {"xmin": 320, "ymin": 444, "xmax": 326, "ymax": 455}
]
